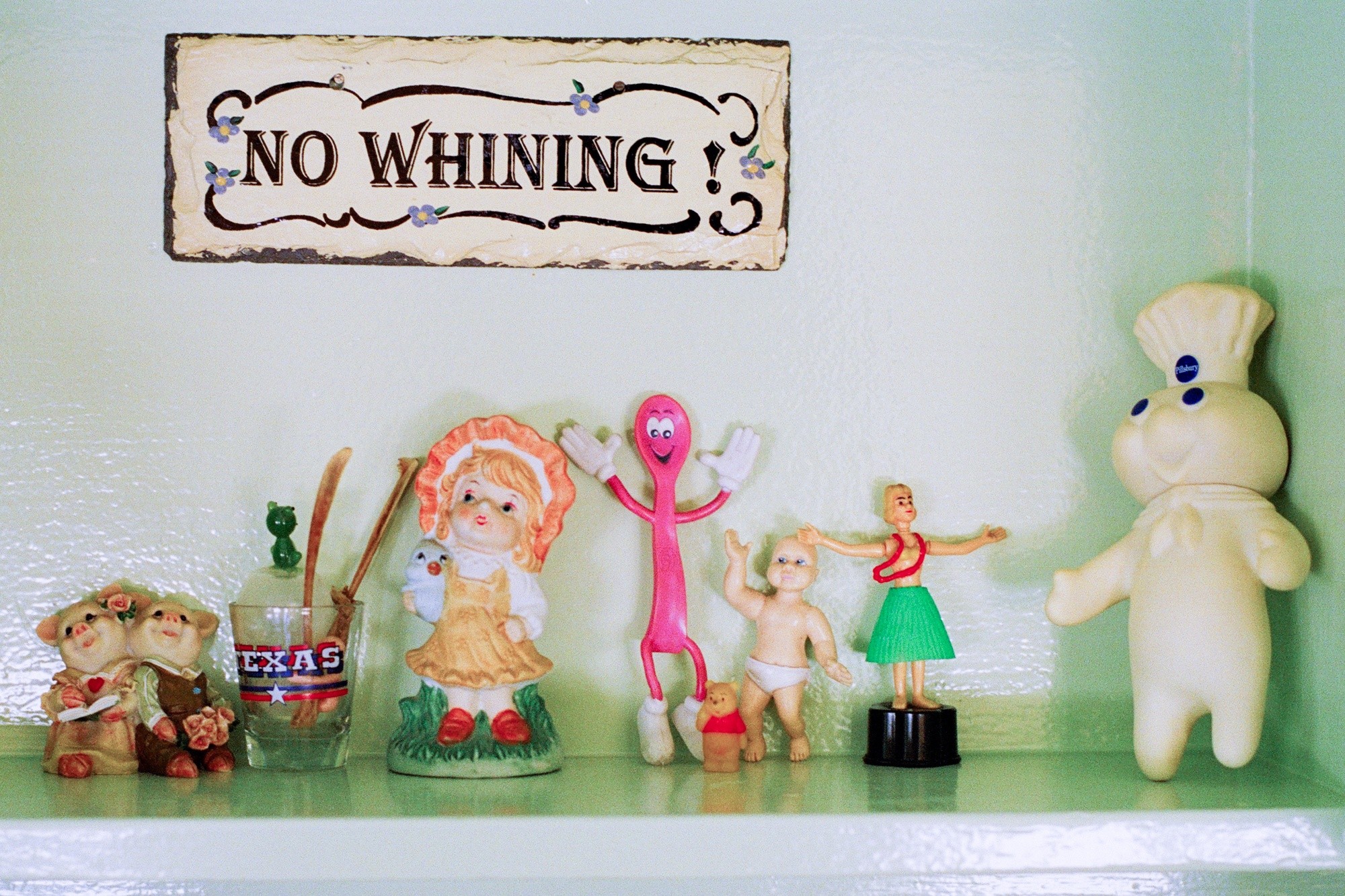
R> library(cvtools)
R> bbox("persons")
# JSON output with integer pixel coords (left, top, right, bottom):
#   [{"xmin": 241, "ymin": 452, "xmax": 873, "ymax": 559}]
[
  {"xmin": 796, "ymin": 483, "xmax": 1007, "ymax": 709},
  {"xmin": 400, "ymin": 415, "xmax": 577, "ymax": 747},
  {"xmin": 723, "ymin": 527, "xmax": 852, "ymax": 762}
]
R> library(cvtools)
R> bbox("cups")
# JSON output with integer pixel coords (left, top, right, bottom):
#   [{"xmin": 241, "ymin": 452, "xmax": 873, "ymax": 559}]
[{"xmin": 228, "ymin": 601, "xmax": 365, "ymax": 770}]
